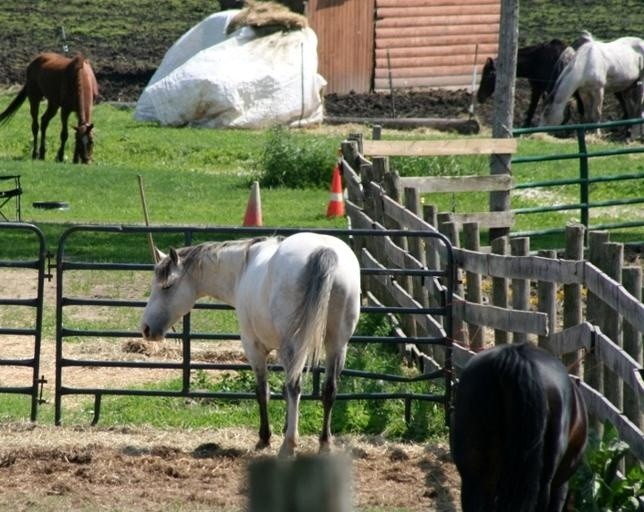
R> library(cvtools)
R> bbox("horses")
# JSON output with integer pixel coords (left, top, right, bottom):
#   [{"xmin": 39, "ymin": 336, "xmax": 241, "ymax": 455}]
[
  {"xmin": 538, "ymin": 36, "xmax": 644, "ymax": 141},
  {"xmin": 0, "ymin": 51, "xmax": 99, "ymax": 164},
  {"xmin": 476, "ymin": 39, "xmax": 634, "ymax": 138},
  {"xmin": 449, "ymin": 341, "xmax": 589, "ymax": 511},
  {"xmin": 141, "ymin": 232, "xmax": 361, "ymax": 462}
]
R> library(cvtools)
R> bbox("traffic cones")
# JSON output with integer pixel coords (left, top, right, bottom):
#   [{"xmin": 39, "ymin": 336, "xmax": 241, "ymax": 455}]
[
  {"xmin": 324, "ymin": 163, "xmax": 345, "ymax": 218},
  {"xmin": 244, "ymin": 182, "xmax": 265, "ymax": 227}
]
